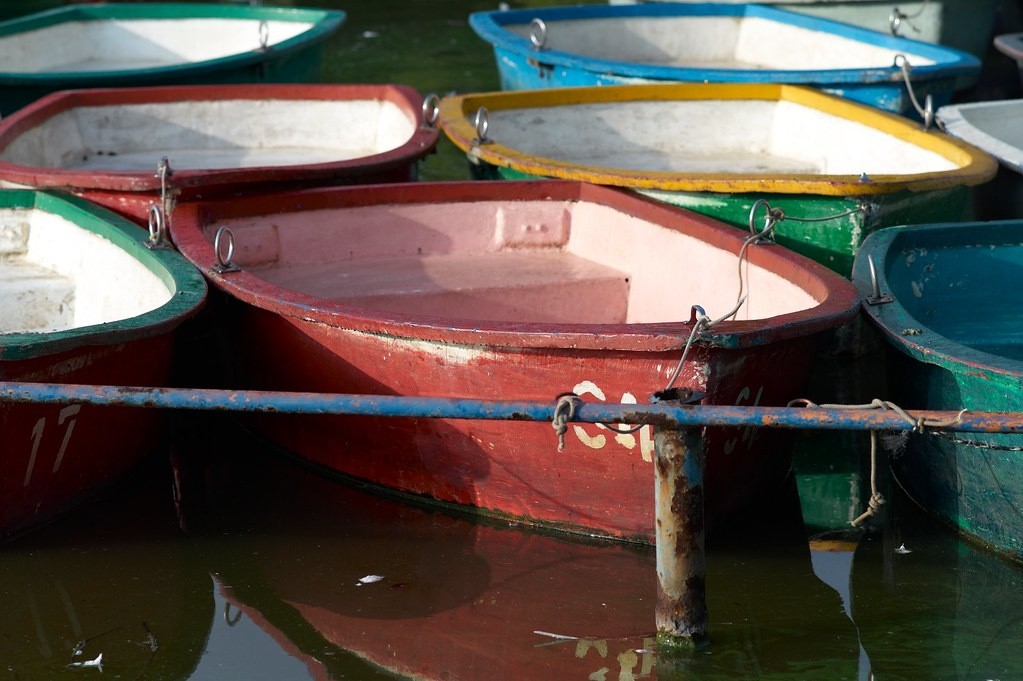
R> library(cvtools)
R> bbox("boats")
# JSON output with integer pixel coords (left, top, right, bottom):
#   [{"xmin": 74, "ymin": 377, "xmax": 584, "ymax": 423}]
[
  {"xmin": 929, "ymin": 95, "xmax": 1022, "ymax": 173},
  {"xmin": 0, "ymin": 85, "xmax": 441, "ymax": 237},
  {"xmin": 0, "ymin": 2, "xmax": 352, "ymax": 111},
  {"xmin": 847, "ymin": 214, "xmax": 1023, "ymax": 571},
  {"xmin": 426, "ymin": 80, "xmax": 1004, "ymax": 290},
  {"xmin": 0, "ymin": 186, "xmax": 216, "ymax": 525},
  {"xmin": 469, "ymin": 5, "xmax": 986, "ymax": 120},
  {"xmin": 777, "ymin": 1, "xmax": 1003, "ymax": 58},
  {"xmin": 161, "ymin": 174, "xmax": 860, "ymax": 551}
]
